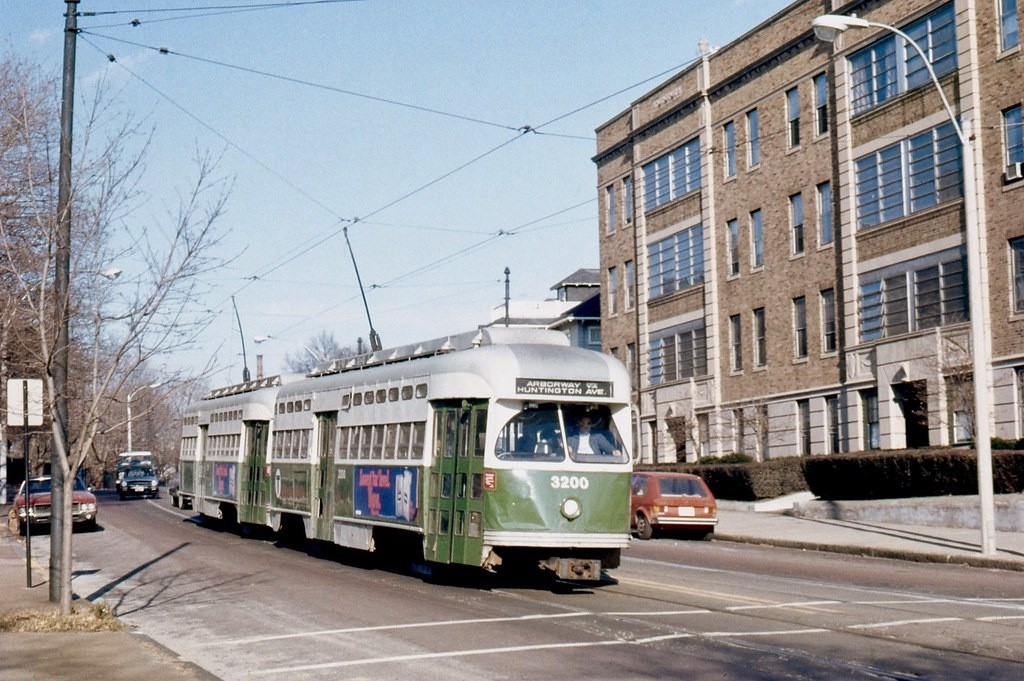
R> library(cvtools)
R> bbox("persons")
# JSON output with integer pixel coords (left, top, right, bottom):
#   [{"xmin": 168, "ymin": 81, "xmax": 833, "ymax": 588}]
[{"xmin": 557, "ymin": 417, "xmax": 621, "ymax": 456}]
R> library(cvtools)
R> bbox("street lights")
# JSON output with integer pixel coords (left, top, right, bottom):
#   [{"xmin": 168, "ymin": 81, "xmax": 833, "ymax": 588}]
[
  {"xmin": 0, "ymin": 267, "xmax": 123, "ymax": 506},
  {"xmin": 126, "ymin": 382, "xmax": 164, "ymax": 452},
  {"xmin": 812, "ymin": 14, "xmax": 997, "ymax": 557}
]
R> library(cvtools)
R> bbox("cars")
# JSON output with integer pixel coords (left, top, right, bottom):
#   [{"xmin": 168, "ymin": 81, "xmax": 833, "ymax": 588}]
[
  {"xmin": 114, "ymin": 450, "xmax": 161, "ymax": 500},
  {"xmin": 12, "ymin": 472, "xmax": 99, "ymax": 536},
  {"xmin": 628, "ymin": 471, "xmax": 718, "ymax": 542}
]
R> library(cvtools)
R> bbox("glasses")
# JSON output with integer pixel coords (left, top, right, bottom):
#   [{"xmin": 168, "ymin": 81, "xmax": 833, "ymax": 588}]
[{"xmin": 580, "ymin": 422, "xmax": 592, "ymax": 427}]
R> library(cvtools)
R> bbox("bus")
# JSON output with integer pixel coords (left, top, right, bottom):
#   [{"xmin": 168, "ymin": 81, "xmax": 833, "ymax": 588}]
[{"xmin": 167, "ymin": 226, "xmax": 646, "ymax": 589}]
